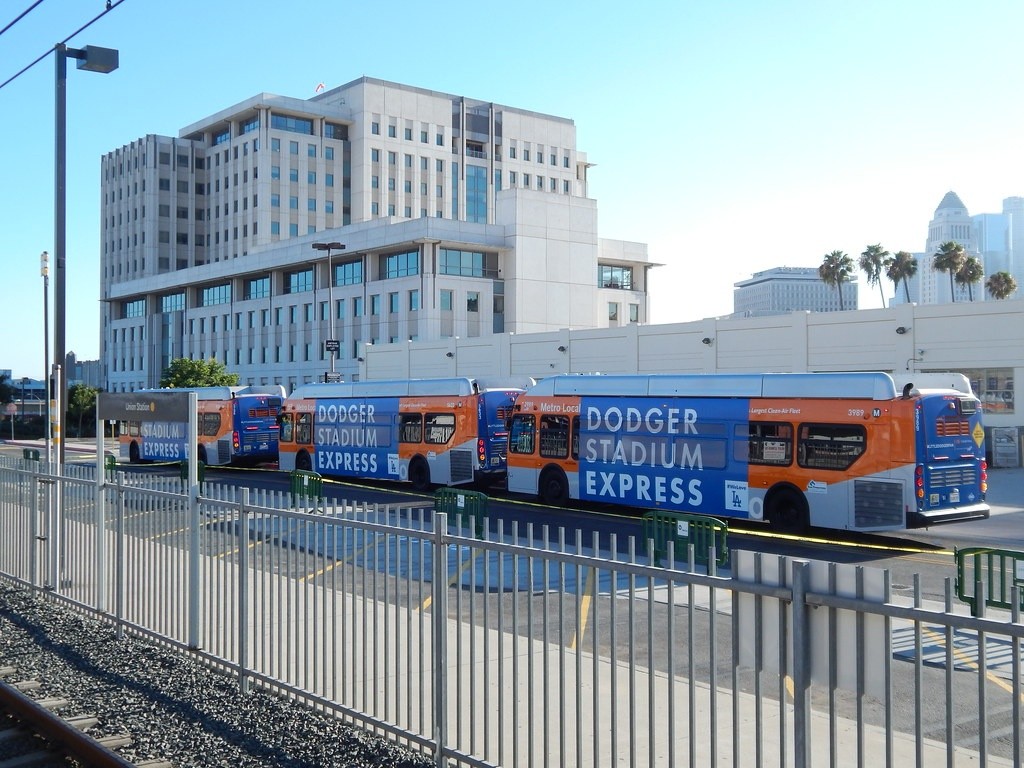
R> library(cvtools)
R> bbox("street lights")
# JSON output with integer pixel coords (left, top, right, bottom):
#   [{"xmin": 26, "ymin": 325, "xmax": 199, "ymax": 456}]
[
  {"xmin": 19, "ymin": 377, "xmax": 31, "ymax": 415},
  {"xmin": 27, "ymin": 394, "xmax": 42, "ymax": 417},
  {"xmin": 50, "ymin": 41, "xmax": 121, "ymax": 591},
  {"xmin": 310, "ymin": 241, "xmax": 346, "ymax": 382}
]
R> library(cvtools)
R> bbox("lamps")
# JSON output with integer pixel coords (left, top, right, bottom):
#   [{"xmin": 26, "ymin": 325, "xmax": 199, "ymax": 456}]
[
  {"xmin": 701, "ymin": 338, "xmax": 711, "ymax": 344},
  {"xmin": 446, "ymin": 352, "xmax": 453, "ymax": 358},
  {"xmin": 895, "ymin": 326, "xmax": 906, "ymax": 335},
  {"xmin": 358, "ymin": 358, "xmax": 364, "ymax": 361},
  {"xmin": 558, "ymin": 346, "xmax": 566, "ymax": 352}
]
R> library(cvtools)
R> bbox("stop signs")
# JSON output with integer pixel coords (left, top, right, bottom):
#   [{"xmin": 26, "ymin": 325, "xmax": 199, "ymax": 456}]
[{"xmin": 8, "ymin": 403, "xmax": 17, "ymax": 415}]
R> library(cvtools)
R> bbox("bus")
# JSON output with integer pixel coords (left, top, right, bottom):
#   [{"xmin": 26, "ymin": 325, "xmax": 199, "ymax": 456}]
[
  {"xmin": 277, "ymin": 376, "xmax": 536, "ymax": 494},
  {"xmin": 119, "ymin": 385, "xmax": 289, "ymax": 470},
  {"xmin": 508, "ymin": 372, "xmax": 992, "ymax": 539}
]
